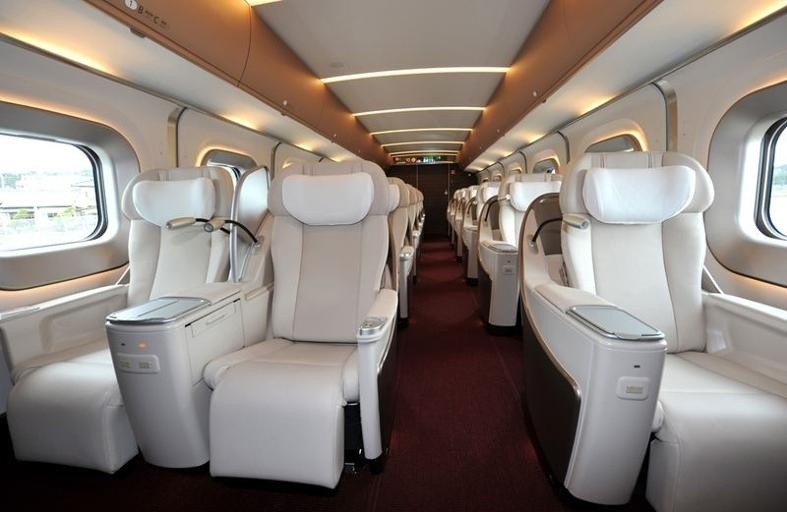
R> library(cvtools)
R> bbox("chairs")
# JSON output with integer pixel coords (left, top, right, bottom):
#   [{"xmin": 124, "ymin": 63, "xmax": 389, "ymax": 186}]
[
  {"xmin": 203, "ymin": 159, "xmax": 398, "ymax": 489},
  {"xmin": 446, "ymin": 173, "xmax": 566, "ymax": 335},
  {"xmin": 388, "ymin": 177, "xmax": 425, "ymax": 329},
  {"xmin": 0, "ymin": 166, "xmax": 240, "ymax": 475},
  {"xmin": 517, "ymin": 152, "xmax": 786, "ymax": 511}
]
